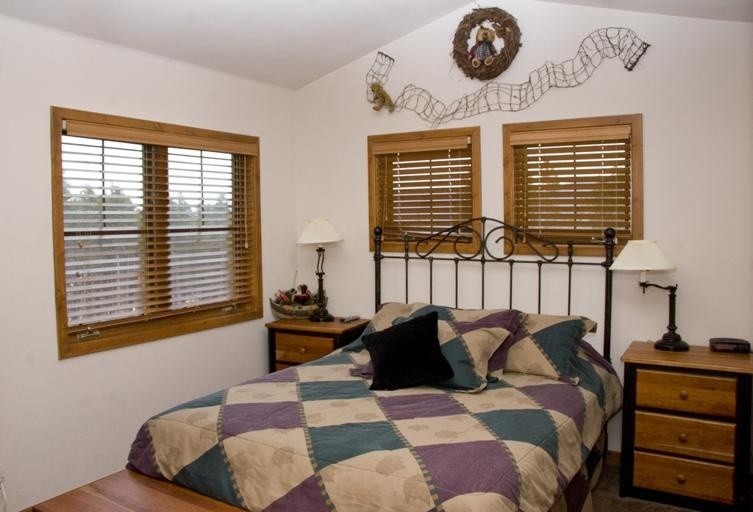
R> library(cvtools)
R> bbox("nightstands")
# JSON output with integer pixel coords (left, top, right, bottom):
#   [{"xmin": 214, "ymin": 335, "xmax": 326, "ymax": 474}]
[
  {"xmin": 263, "ymin": 313, "xmax": 369, "ymax": 377},
  {"xmin": 614, "ymin": 339, "xmax": 752, "ymax": 512}
]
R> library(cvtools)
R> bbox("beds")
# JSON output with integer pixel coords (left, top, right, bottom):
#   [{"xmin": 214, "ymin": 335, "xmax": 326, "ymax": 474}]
[{"xmin": 129, "ymin": 216, "xmax": 627, "ymax": 511}]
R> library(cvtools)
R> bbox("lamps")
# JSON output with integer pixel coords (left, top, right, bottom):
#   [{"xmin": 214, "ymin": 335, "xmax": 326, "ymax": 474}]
[
  {"xmin": 606, "ymin": 238, "xmax": 694, "ymax": 352},
  {"xmin": 294, "ymin": 219, "xmax": 345, "ymax": 325}
]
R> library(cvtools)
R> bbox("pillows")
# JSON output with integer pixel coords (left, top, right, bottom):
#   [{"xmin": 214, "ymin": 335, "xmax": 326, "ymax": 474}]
[
  {"xmin": 500, "ymin": 306, "xmax": 599, "ymax": 387},
  {"xmin": 484, "ymin": 330, "xmax": 516, "ymax": 389},
  {"xmin": 359, "ymin": 308, "xmax": 450, "ymax": 393},
  {"xmin": 341, "ymin": 295, "xmax": 419, "ymax": 355},
  {"xmin": 352, "ymin": 300, "xmax": 525, "ymax": 395}
]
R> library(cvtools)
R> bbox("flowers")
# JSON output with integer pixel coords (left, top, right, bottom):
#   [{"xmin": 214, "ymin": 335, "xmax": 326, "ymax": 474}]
[{"xmin": 273, "ymin": 286, "xmax": 322, "ymax": 307}]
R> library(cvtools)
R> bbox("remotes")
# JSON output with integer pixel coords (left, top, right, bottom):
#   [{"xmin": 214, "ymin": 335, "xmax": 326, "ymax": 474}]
[{"xmin": 343, "ymin": 315, "xmax": 360, "ymax": 323}]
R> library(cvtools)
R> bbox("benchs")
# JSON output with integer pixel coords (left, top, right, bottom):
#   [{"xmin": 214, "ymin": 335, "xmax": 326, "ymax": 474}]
[{"xmin": 30, "ymin": 469, "xmax": 247, "ymax": 512}]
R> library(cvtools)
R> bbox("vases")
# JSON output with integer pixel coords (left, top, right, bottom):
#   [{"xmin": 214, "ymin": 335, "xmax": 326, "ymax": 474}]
[{"xmin": 268, "ymin": 297, "xmax": 327, "ymax": 320}]
